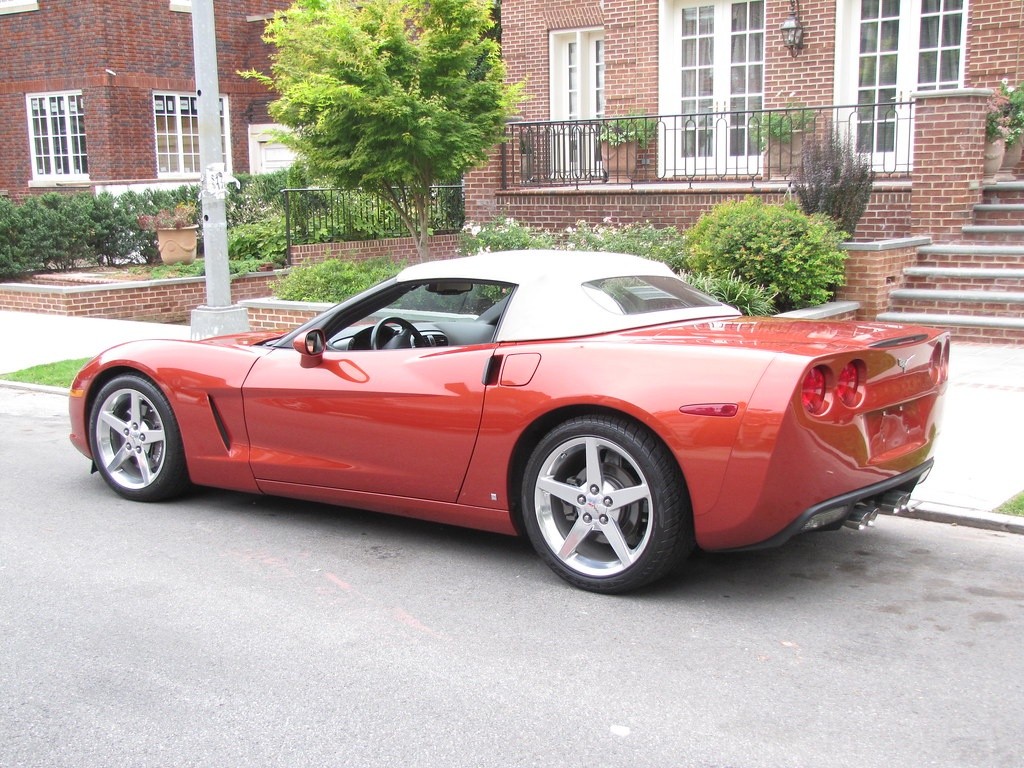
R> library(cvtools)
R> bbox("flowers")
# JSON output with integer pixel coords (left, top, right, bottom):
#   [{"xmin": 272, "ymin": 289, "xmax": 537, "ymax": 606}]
[{"xmin": 985, "ymin": 77, "xmax": 1024, "ymax": 148}]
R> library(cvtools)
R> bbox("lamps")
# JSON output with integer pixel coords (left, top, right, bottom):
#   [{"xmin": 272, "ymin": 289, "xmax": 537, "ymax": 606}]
[{"xmin": 781, "ymin": 0, "xmax": 804, "ymax": 59}]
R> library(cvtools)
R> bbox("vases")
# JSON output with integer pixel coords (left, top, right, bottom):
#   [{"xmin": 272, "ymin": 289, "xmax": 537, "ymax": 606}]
[{"xmin": 983, "ymin": 132, "xmax": 1024, "ymax": 185}]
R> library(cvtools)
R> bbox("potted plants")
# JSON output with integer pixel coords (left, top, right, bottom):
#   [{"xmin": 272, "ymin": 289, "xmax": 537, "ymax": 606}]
[
  {"xmin": 520, "ymin": 139, "xmax": 534, "ymax": 180},
  {"xmin": 748, "ymin": 91, "xmax": 817, "ymax": 180},
  {"xmin": 139, "ymin": 203, "xmax": 201, "ymax": 266},
  {"xmin": 594, "ymin": 111, "xmax": 659, "ymax": 184}
]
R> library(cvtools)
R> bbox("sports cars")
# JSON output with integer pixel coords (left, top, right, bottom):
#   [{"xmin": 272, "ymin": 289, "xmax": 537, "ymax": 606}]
[{"xmin": 67, "ymin": 247, "xmax": 952, "ymax": 595}]
